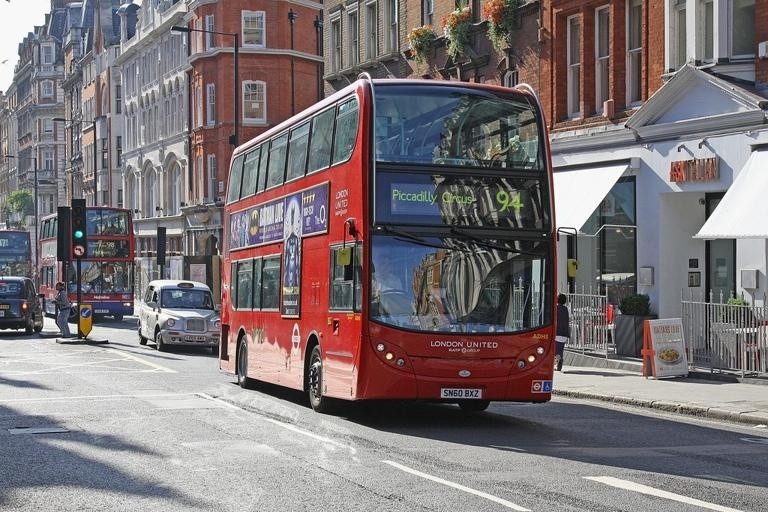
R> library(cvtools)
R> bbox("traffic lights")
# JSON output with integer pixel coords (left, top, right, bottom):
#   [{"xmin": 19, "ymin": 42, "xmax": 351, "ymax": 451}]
[{"xmin": 71, "ymin": 198, "xmax": 88, "ymax": 258}]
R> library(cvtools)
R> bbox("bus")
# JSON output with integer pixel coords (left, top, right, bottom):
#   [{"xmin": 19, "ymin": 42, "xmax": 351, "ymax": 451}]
[
  {"xmin": 38, "ymin": 205, "xmax": 137, "ymax": 322},
  {"xmin": 218, "ymin": 69, "xmax": 582, "ymax": 415},
  {"xmin": 0, "ymin": 223, "xmax": 33, "ymax": 278}
]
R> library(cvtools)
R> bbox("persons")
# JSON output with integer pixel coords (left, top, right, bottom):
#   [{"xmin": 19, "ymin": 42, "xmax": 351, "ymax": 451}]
[
  {"xmin": 48, "ymin": 281, "xmax": 74, "ymax": 339},
  {"xmin": 555, "ymin": 291, "xmax": 570, "ymax": 373}
]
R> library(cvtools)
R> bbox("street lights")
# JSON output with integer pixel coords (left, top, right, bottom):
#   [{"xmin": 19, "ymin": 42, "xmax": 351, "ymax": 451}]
[
  {"xmin": 5, "ymin": 154, "xmax": 38, "ymax": 294},
  {"xmin": 169, "ymin": 25, "xmax": 239, "ymax": 146},
  {"xmin": 52, "ymin": 116, "xmax": 97, "ymax": 207}
]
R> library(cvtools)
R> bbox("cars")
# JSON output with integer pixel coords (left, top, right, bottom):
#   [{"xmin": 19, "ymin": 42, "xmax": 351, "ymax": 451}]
[
  {"xmin": 0, "ymin": 275, "xmax": 44, "ymax": 335},
  {"xmin": 137, "ymin": 277, "xmax": 222, "ymax": 355}
]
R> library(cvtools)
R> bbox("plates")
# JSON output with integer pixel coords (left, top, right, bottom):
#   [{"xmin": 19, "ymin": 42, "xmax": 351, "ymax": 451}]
[{"xmin": 656, "ymin": 345, "xmax": 683, "ymax": 362}]
